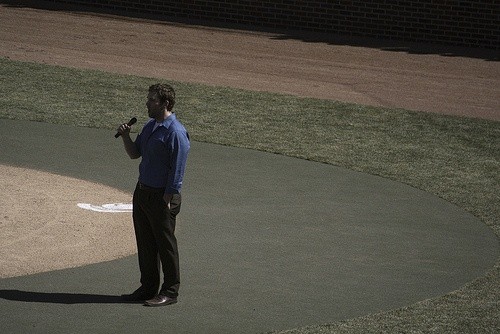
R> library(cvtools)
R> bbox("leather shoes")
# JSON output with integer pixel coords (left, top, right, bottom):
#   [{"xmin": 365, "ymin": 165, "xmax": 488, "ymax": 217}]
[
  {"xmin": 144, "ymin": 294, "xmax": 177, "ymax": 307},
  {"xmin": 121, "ymin": 287, "xmax": 159, "ymax": 301}
]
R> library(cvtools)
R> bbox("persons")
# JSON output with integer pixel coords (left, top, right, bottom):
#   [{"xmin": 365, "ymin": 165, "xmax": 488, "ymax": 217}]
[{"xmin": 118, "ymin": 83, "xmax": 190, "ymax": 306}]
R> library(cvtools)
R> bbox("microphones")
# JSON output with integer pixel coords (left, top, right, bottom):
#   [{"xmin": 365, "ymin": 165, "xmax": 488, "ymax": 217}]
[{"xmin": 115, "ymin": 118, "xmax": 137, "ymax": 138}]
